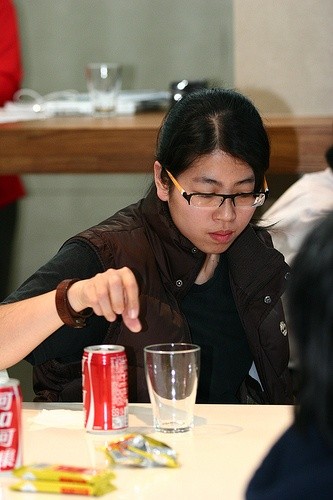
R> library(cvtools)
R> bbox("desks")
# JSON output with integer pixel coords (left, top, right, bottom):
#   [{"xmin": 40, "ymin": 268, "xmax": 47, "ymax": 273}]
[
  {"xmin": 0, "ymin": 115, "xmax": 333, "ymax": 171},
  {"xmin": 0, "ymin": 403, "xmax": 296, "ymax": 500}
]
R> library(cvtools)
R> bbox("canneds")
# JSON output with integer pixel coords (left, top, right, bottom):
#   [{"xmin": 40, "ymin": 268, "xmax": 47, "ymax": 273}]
[
  {"xmin": 81, "ymin": 344, "xmax": 130, "ymax": 434},
  {"xmin": 0, "ymin": 378, "xmax": 23, "ymax": 475}
]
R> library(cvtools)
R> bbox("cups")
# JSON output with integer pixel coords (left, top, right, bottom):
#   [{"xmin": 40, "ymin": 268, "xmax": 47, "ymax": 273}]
[
  {"xmin": 85, "ymin": 63, "xmax": 123, "ymax": 118},
  {"xmin": 145, "ymin": 344, "xmax": 201, "ymax": 434},
  {"xmin": 171, "ymin": 82, "xmax": 207, "ymax": 105}
]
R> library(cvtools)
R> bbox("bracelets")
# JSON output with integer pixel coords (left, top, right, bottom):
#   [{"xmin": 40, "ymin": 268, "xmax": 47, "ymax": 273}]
[{"xmin": 55, "ymin": 278, "xmax": 94, "ymax": 329}]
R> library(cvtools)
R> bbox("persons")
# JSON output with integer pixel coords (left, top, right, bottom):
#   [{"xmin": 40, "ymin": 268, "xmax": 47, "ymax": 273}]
[
  {"xmin": 0, "ymin": 0, "xmax": 27, "ymax": 301},
  {"xmin": 0, "ymin": 87, "xmax": 294, "ymax": 405},
  {"xmin": 244, "ymin": 144, "xmax": 333, "ymax": 499}
]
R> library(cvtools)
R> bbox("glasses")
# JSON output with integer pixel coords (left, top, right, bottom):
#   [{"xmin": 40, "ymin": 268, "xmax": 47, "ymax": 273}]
[{"xmin": 165, "ymin": 170, "xmax": 269, "ymax": 209}]
